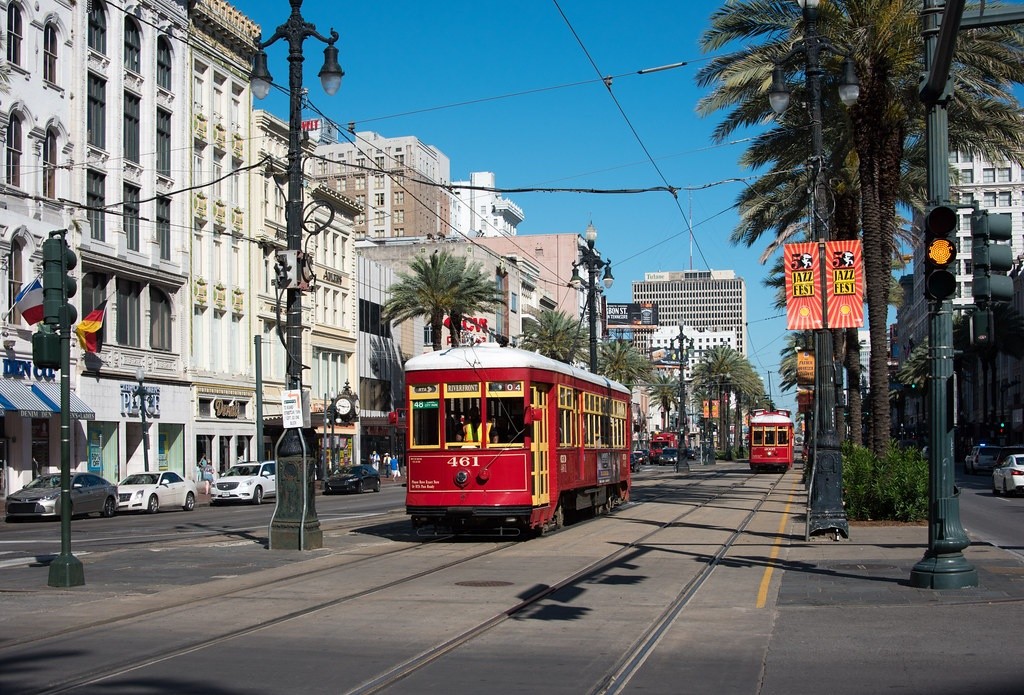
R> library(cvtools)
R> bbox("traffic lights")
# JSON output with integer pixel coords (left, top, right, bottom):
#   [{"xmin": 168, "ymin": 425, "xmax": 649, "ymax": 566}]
[
  {"xmin": 970, "ymin": 208, "xmax": 1017, "ymax": 304},
  {"xmin": 31, "ymin": 228, "xmax": 78, "ymax": 368},
  {"xmin": 922, "ymin": 207, "xmax": 961, "ymax": 300}
]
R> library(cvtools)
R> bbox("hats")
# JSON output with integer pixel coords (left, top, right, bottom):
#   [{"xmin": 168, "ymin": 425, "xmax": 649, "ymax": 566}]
[
  {"xmin": 373, "ymin": 451, "xmax": 376, "ymax": 454},
  {"xmin": 385, "ymin": 453, "xmax": 390, "ymax": 455}
]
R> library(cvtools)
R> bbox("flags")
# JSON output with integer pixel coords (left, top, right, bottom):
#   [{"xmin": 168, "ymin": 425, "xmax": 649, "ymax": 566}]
[
  {"xmin": 14, "ymin": 279, "xmax": 45, "ymax": 326},
  {"xmin": 74, "ymin": 299, "xmax": 107, "ymax": 354}
]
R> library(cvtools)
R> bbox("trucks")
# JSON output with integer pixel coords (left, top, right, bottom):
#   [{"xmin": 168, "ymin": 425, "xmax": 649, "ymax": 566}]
[{"xmin": 649, "ymin": 434, "xmax": 678, "ymax": 465}]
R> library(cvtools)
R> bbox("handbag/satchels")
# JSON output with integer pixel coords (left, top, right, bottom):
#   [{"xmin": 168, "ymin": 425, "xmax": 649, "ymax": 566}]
[{"xmin": 398, "ymin": 471, "xmax": 401, "ymax": 477}]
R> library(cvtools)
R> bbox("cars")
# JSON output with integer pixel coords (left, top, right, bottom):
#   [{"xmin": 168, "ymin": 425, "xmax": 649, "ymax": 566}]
[
  {"xmin": 687, "ymin": 450, "xmax": 696, "ymax": 460},
  {"xmin": 632, "ymin": 451, "xmax": 646, "ymax": 465},
  {"xmin": 630, "ymin": 453, "xmax": 640, "ymax": 472},
  {"xmin": 116, "ymin": 470, "xmax": 198, "ymax": 514},
  {"xmin": 5, "ymin": 472, "xmax": 120, "ymax": 521},
  {"xmin": 990, "ymin": 454, "xmax": 1024, "ymax": 497},
  {"xmin": 325, "ymin": 465, "xmax": 381, "ymax": 495}
]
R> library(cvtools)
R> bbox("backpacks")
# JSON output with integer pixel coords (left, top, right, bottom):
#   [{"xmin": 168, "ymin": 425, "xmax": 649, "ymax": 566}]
[{"xmin": 203, "ymin": 467, "xmax": 213, "ymax": 481}]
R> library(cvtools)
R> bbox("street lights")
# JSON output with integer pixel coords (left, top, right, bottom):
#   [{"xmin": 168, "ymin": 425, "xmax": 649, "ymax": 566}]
[
  {"xmin": 129, "ymin": 367, "xmax": 155, "ymax": 472},
  {"xmin": 669, "ymin": 318, "xmax": 695, "ymax": 472},
  {"xmin": 245, "ymin": 0, "xmax": 347, "ymax": 551},
  {"xmin": 569, "ymin": 219, "xmax": 615, "ymax": 377},
  {"xmin": 323, "ymin": 387, "xmax": 342, "ymax": 471}
]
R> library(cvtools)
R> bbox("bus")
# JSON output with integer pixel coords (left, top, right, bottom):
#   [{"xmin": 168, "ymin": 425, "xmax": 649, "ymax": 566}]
[
  {"xmin": 747, "ymin": 409, "xmax": 795, "ymax": 473},
  {"xmin": 404, "ymin": 346, "xmax": 632, "ymax": 537}
]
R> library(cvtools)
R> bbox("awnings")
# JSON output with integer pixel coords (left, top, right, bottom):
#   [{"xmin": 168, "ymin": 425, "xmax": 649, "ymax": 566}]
[{"xmin": 0, "ymin": 379, "xmax": 95, "ymax": 419}]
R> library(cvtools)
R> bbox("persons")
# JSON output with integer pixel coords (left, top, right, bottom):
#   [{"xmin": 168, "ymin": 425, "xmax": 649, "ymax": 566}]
[
  {"xmin": 199, "ymin": 454, "xmax": 207, "ymax": 480},
  {"xmin": 204, "ymin": 459, "xmax": 215, "ymax": 495},
  {"xmin": 455, "ymin": 406, "xmax": 499, "ymax": 443},
  {"xmin": 370, "ymin": 451, "xmax": 399, "ymax": 482}
]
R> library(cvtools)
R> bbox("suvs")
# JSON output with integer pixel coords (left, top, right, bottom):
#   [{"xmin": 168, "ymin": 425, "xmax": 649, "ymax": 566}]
[
  {"xmin": 964, "ymin": 443, "xmax": 1001, "ymax": 475},
  {"xmin": 658, "ymin": 448, "xmax": 679, "ymax": 466},
  {"xmin": 210, "ymin": 459, "xmax": 277, "ymax": 505},
  {"xmin": 992, "ymin": 445, "xmax": 1024, "ymax": 471}
]
